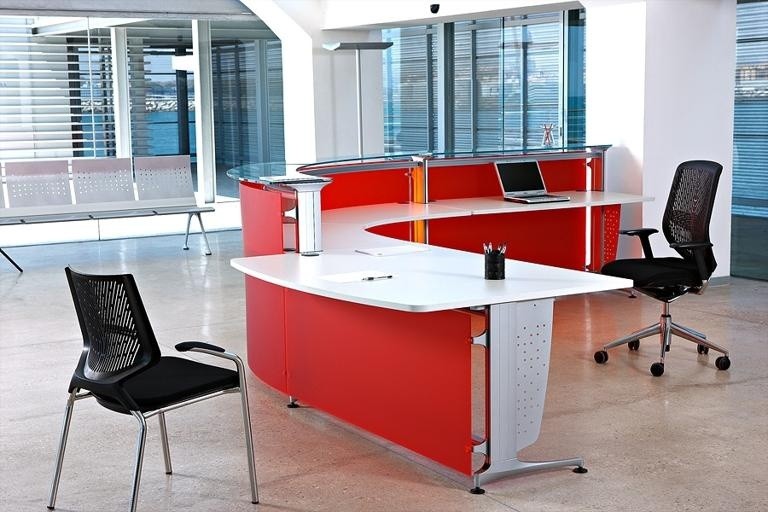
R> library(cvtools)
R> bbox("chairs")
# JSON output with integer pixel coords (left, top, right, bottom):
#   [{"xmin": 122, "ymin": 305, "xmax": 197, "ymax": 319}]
[
  {"xmin": 47, "ymin": 268, "xmax": 258, "ymax": 512},
  {"xmin": 593, "ymin": 160, "xmax": 732, "ymax": 376}
]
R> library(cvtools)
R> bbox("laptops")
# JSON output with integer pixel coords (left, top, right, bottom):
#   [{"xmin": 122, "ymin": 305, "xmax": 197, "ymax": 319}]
[{"xmin": 493, "ymin": 158, "xmax": 570, "ymax": 204}]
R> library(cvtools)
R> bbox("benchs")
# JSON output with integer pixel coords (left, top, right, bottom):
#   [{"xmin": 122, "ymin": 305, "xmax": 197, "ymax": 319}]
[{"xmin": 1, "ymin": 153, "xmax": 215, "ymax": 274}]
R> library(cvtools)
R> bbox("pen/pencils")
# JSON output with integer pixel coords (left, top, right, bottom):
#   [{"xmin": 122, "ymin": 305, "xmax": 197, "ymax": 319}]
[
  {"xmin": 542, "ymin": 123, "xmax": 554, "ymax": 145},
  {"xmin": 361, "ymin": 276, "xmax": 391, "ymax": 281},
  {"xmin": 482, "ymin": 241, "xmax": 508, "ymax": 255}
]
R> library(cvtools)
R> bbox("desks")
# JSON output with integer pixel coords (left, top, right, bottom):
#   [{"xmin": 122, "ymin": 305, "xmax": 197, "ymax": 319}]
[{"xmin": 224, "ymin": 146, "xmax": 656, "ymax": 498}]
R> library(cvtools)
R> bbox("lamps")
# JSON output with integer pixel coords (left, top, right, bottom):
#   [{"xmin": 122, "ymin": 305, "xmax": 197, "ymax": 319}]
[{"xmin": 322, "ymin": 41, "xmax": 394, "ymax": 155}]
